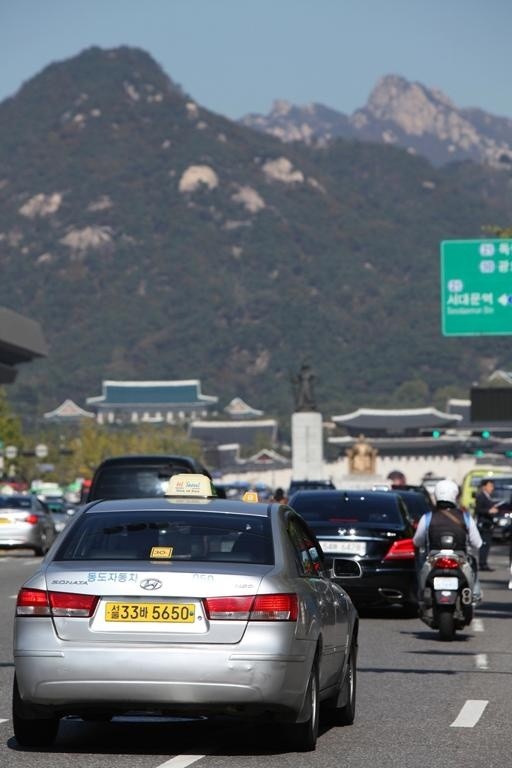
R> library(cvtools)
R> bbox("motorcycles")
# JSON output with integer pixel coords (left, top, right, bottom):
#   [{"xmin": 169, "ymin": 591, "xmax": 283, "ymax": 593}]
[{"xmin": 410, "ymin": 534, "xmax": 489, "ymax": 642}]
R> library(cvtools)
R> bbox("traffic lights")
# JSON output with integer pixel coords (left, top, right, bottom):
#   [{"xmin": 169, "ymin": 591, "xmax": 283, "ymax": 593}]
[
  {"xmin": 420, "ymin": 430, "xmax": 446, "ymax": 437},
  {"xmin": 470, "ymin": 430, "xmax": 492, "ymax": 439}
]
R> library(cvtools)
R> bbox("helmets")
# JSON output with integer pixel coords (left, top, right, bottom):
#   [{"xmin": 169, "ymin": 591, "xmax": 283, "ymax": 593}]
[{"xmin": 434, "ymin": 479, "xmax": 461, "ymax": 507}]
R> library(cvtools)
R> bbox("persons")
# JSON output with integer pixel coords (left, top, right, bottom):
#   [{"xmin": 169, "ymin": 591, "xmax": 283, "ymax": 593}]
[
  {"xmin": 475, "ymin": 478, "xmax": 499, "ymax": 572},
  {"xmin": 266, "ymin": 488, "xmax": 288, "ymax": 504},
  {"xmin": 413, "ymin": 479, "xmax": 483, "ymax": 581}
]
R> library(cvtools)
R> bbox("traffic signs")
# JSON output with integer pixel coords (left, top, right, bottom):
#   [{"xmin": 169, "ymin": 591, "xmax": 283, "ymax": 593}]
[{"xmin": 439, "ymin": 236, "xmax": 512, "ymax": 338}]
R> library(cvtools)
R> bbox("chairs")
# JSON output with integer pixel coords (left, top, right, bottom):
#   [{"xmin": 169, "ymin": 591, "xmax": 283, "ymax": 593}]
[
  {"xmin": 231, "ymin": 531, "xmax": 274, "ymax": 561},
  {"xmin": 119, "ymin": 528, "xmax": 162, "ymax": 556}
]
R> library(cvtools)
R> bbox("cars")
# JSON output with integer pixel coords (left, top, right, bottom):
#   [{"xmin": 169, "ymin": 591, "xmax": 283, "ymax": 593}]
[{"xmin": 12, "ymin": 495, "xmax": 360, "ymax": 750}]
